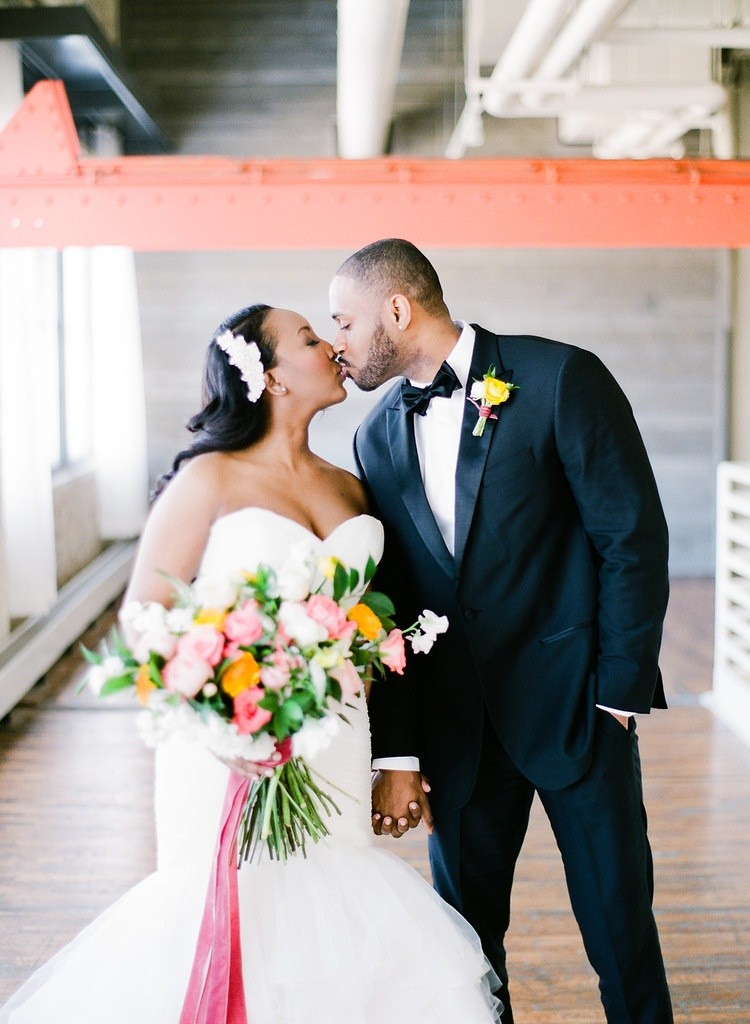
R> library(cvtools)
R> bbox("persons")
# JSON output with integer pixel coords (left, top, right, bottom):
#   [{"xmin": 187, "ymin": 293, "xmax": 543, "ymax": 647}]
[
  {"xmin": 327, "ymin": 237, "xmax": 674, "ymax": 1024},
  {"xmin": 0, "ymin": 303, "xmax": 506, "ymax": 1024}
]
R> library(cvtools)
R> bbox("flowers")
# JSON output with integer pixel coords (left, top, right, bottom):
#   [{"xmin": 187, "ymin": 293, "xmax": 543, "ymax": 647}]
[
  {"xmin": 84, "ymin": 557, "xmax": 452, "ymax": 867},
  {"xmin": 465, "ymin": 365, "xmax": 522, "ymax": 437},
  {"xmin": 217, "ymin": 331, "xmax": 269, "ymax": 403}
]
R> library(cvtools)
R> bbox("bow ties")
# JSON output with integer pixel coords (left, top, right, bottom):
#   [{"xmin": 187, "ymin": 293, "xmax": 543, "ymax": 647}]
[{"xmin": 401, "ymin": 360, "xmax": 462, "ymax": 417}]
